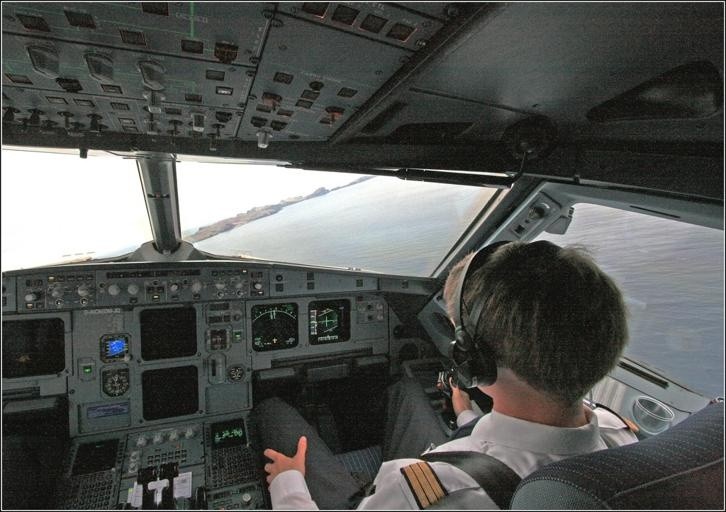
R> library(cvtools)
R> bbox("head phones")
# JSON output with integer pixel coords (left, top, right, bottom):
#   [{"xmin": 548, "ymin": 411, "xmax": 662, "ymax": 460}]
[{"xmin": 449, "ymin": 239, "xmax": 561, "ymax": 391}]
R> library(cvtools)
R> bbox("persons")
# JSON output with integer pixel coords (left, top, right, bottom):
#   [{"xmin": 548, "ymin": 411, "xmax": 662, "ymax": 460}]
[{"xmin": 247, "ymin": 241, "xmax": 640, "ymax": 509}]
